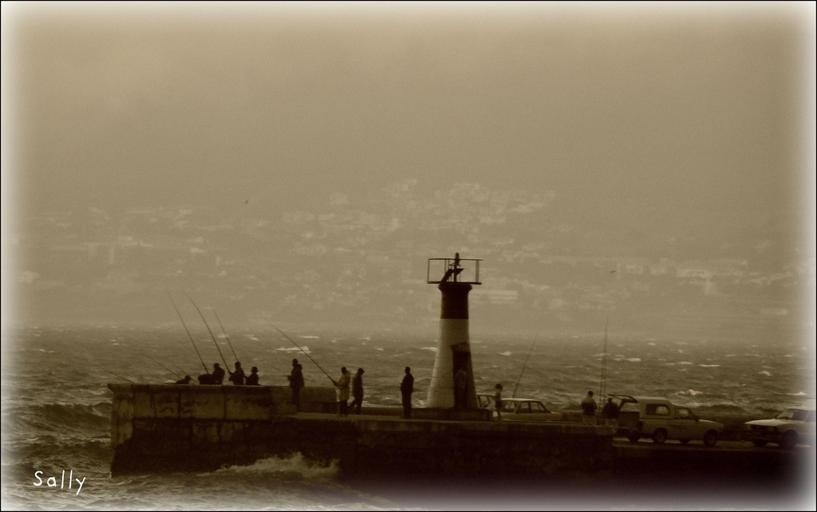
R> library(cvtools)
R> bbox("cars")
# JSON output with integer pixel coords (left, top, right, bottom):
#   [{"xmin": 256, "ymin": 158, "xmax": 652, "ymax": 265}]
[
  {"xmin": 744, "ymin": 404, "xmax": 816, "ymax": 451},
  {"xmin": 477, "ymin": 392, "xmax": 497, "ymax": 410},
  {"xmin": 608, "ymin": 392, "xmax": 725, "ymax": 448},
  {"xmin": 486, "ymin": 397, "xmax": 562, "ymax": 422}
]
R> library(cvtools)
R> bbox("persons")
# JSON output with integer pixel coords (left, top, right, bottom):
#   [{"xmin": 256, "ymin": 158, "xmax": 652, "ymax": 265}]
[
  {"xmin": 581, "ymin": 391, "xmax": 597, "ymax": 416},
  {"xmin": 212, "ymin": 363, "xmax": 225, "ymax": 384},
  {"xmin": 491, "ymin": 384, "xmax": 505, "ymax": 420},
  {"xmin": 400, "ymin": 367, "xmax": 414, "ymax": 418},
  {"xmin": 350, "ymin": 368, "xmax": 364, "ymax": 415},
  {"xmin": 288, "ymin": 359, "xmax": 304, "ymax": 411},
  {"xmin": 602, "ymin": 398, "xmax": 620, "ymax": 418},
  {"xmin": 229, "ymin": 362, "xmax": 244, "ymax": 385},
  {"xmin": 334, "ymin": 367, "xmax": 351, "ymax": 415},
  {"xmin": 176, "ymin": 375, "xmax": 191, "ymax": 385},
  {"xmin": 245, "ymin": 367, "xmax": 259, "ymax": 385}
]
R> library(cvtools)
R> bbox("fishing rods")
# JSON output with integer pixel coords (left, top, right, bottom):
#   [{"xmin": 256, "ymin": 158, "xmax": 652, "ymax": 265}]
[
  {"xmin": 190, "ymin": 300, "xmax": 231, "ymax": 376},
  {"xmin": 505, "ymin": 335, "xmax": 538, "ymax": 410},
  {"xmin": 277, "ymin": 332, "xmax": 338, "ymax": 388},
  {"xmin": 215, "ymin": 308, "xmax": 244, "ymax": 376},
  {"xmin": 174, "ymin": 308, "xmax": 208, "ymax": 375},
  {"xmin": 595, "ymin": 338, "xmax": 608, "ymax": 416}
]
